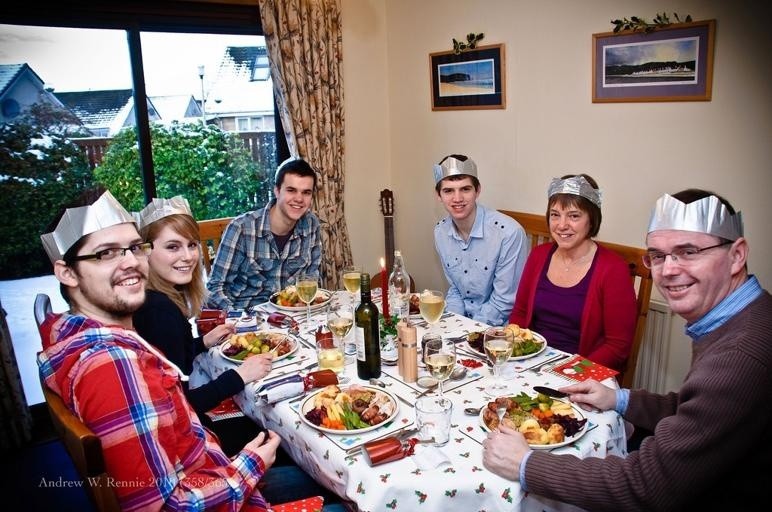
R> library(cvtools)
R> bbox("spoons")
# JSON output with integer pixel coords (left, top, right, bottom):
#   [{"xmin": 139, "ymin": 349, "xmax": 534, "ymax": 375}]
[{"xmin": 416, "ymin": 366, "xmax": 468, "ymax": 399}]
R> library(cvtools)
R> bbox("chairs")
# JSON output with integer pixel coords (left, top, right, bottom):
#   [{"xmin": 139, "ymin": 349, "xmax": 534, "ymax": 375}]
[
  {"xmin": 593, "ymin": 242, "xmax": 654, "ymax": 388},
  {"xmin": 497, "ymin": 206, "xmax": 558, "ymax": 256},
  {"xmin": 35, "ymin": 293, "xmax": 53, "ymax": 340},
  {"xmin": 198, "ymin": 218, "xmax": 236, "ymax": 276},
  {"xmin": 36, "ymin": 369, "xmax": 124, "ymax": 511}
]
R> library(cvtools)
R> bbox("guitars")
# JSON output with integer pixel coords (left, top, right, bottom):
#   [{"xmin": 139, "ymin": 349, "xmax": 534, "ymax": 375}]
[{"xmin": 370, "ymin": 190, "xmax": 415, "ymax": 292}]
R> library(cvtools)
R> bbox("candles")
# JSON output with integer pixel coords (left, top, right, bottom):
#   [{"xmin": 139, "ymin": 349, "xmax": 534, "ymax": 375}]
[{"xmin": 380, "ymin": 257, "xmax": 389, "ymax": 323}]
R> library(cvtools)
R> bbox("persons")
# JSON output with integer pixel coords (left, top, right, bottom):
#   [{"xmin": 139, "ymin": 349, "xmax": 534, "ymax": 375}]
[
  {"xmin": 205, "ymin": 156, "xmax": 323, "ymax": 315},
  {"xmin": 482, "ymin": 187, "xmax": 771, "ymax": 511},
  {"xmin": 35, "ymin": 189, "xmax": 348, "ymax": 510},
  {"xmin": 508, "ymin": 173, "xmax": 641, "ymax": 386},
  {"xmin": 126, "ymin": 193, "xmax": 295, "ymax": 465},
  {"xmin": 432, "ymin": 153, "xmax": 531, "ymax": 330}
]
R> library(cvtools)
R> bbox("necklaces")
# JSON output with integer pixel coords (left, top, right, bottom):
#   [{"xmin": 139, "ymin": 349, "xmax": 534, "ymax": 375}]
[{"xmin": 556, "ymin": 252, "xmax": 586, "ymax": 272}]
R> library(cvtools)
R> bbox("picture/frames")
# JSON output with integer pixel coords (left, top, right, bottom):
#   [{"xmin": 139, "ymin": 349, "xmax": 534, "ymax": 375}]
[
  {"xmin": 592, "ymin": 19, "xmax": 716, "ymax": 103},
  {"xmin": 428, "ymin": 44, "xmax": 507, "ymax": 112}
]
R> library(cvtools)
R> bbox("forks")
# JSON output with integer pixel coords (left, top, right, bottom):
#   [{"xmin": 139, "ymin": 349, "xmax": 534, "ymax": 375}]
[{"xmin": 526, "ymin": 356, "xmax": 569, "ymax": 372}]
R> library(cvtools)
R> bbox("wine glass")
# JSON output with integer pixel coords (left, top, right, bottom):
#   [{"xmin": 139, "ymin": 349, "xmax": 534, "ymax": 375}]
[
  {"xmin": 483, "ymin": 327, "xmax": 514, "ymax": 398},
  {"xmin": 296, "ymin": 275, "xmax": 318, "ymax": 327},
  {"xmin": 327, "ymin": 304, "xmax": 354, "ymax": 365},
  {"xmin": 424, "ymin": 339, "xmax": 456, "ymax": 396},
  {"xmin": 342, "ymin": 265, "xmax": 363, "ymax": 312},
  {"xmin": 419, "ymin": 291, "xmax": 446, "ymax": 333}
]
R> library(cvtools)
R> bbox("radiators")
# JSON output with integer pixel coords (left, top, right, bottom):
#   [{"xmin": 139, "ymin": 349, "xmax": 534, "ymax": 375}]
[{"xmin": 631, "ymin": 298, "xmax": 673, "ymax": 395}]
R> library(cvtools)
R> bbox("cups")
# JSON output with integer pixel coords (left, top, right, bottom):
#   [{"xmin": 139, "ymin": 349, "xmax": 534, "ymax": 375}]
[
  {"xmin": 415, "ymin": 396, "xmax": 452, "ymax": 448},
  {"xmin": 421, "ymin": 335, "xmax": 442, "ymax": 364},
  {"xmin": 316, "ymin": 338, "xmax": 344, "ymax": 377}
]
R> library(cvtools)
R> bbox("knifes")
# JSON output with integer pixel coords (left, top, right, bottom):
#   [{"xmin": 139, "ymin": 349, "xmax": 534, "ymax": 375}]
[
  {"xmin": 270, "ymin": 325, "xmax": 297, "ymax": 353},
  {"xmin": 532, "ymin": 386, "xmax": 570, "ymax": 398}
]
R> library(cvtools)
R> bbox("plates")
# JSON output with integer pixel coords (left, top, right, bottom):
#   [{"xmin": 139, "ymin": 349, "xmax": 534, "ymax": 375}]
[
  {"xmin": 478, "ymin": 395, "xmax": 589, "ymax": 450},
  {"xmin": 466, "ymin": 326, "xmax": 547, "ymax": 361},
  {"xmin": 269, "ymin": 287, "xmax": 333, "ymax": 311},
  {"xmin": 219, "ymin": 334, "xmax": 299, "ymax": 363},
  {"xmin": 299, "ymin": 384, "xmax": 399, "ymax": 434}
]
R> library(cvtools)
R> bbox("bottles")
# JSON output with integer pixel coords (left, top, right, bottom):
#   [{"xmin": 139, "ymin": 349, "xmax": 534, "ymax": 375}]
[
  {"xmin": 388, "ymin": 251, "xmax": 411, "ymax": 323},
  {"xmin": 355, "ymin": 273, "xmax": 382, "ymax": 380}
]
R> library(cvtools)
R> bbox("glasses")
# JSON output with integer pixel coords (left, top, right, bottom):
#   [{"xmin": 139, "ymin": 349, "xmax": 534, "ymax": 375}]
[
  {"xmin": 75, "ymin": 242, "xmax": 153, "ymax": 264},
  {"xmin": 641, "ymin": 242, "xmax": 733, "ymax": 269}
]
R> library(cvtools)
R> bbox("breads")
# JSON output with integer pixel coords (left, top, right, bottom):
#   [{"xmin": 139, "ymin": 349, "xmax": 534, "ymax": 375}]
[
  {"xmin": 548, "ymin": 424, "xmax": 564, "ymax": 444},
  {"xmin": 505, "ymin": 323, "xmax": 520, "ymax": 336},
  {"xmin": 520, "ymin": 428, "xmax": 548, "ymax": 445},
  {"xmin": 519, "ymin": 329, "xmax": 533, "ymax": 340},
  {"xmin": 315, "ymin": 392, "xmax": 329, "ymax": 407},
  {"xmin": 334, "ymin": 392, "xmax": 353, "ymax": 402},
  {"xmin": 323, "ymin": 385, "xmax": 341, "ymax": 400},
  {"xmin": 520, "ymin": 419, "xmax": 540, "ymax": 432}
]
